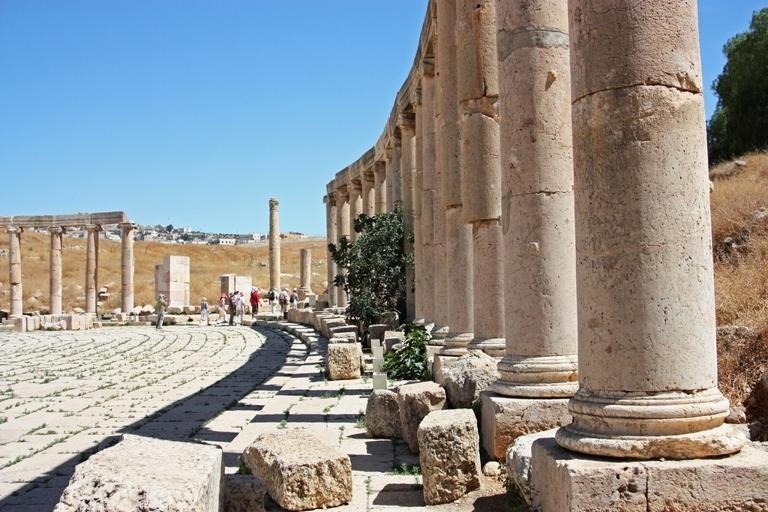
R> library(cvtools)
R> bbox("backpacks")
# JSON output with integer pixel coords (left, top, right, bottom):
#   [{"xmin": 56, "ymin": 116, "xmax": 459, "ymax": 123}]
[{"xmin": 290, "ymin": 294, "xmax": 295, "ymax": 303}]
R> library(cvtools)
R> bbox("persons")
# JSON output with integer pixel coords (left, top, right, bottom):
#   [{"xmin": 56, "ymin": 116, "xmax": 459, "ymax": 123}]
[
  {"xmin": 156, "ymin": 294, "xmax": 167, "ymax": 329},
  {"xmin": 198, "ymin": 287, "xmax": 299, "ymax": 326}
]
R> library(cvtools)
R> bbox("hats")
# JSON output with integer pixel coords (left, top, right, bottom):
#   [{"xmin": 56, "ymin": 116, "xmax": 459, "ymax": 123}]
[
  {"xmin": 221, "ymin": 293, "xmax": 227, "ymax": 297},
  {"xmin": 202, "ymin": 297, "xmax": 208, "ymax": 301},
  {"xmin": 239, "ymin": 292, "xmax": 244, "ymax": 296}
]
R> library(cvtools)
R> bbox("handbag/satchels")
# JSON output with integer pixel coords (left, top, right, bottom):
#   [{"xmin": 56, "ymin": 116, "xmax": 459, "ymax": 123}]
[
  {"xmin": 219, "ymin": 311, "xmax": 225, "ymax": 317},
  {"xmin": 269, "ymin": 292, "xmax": 274, "ymax": 300}
]
